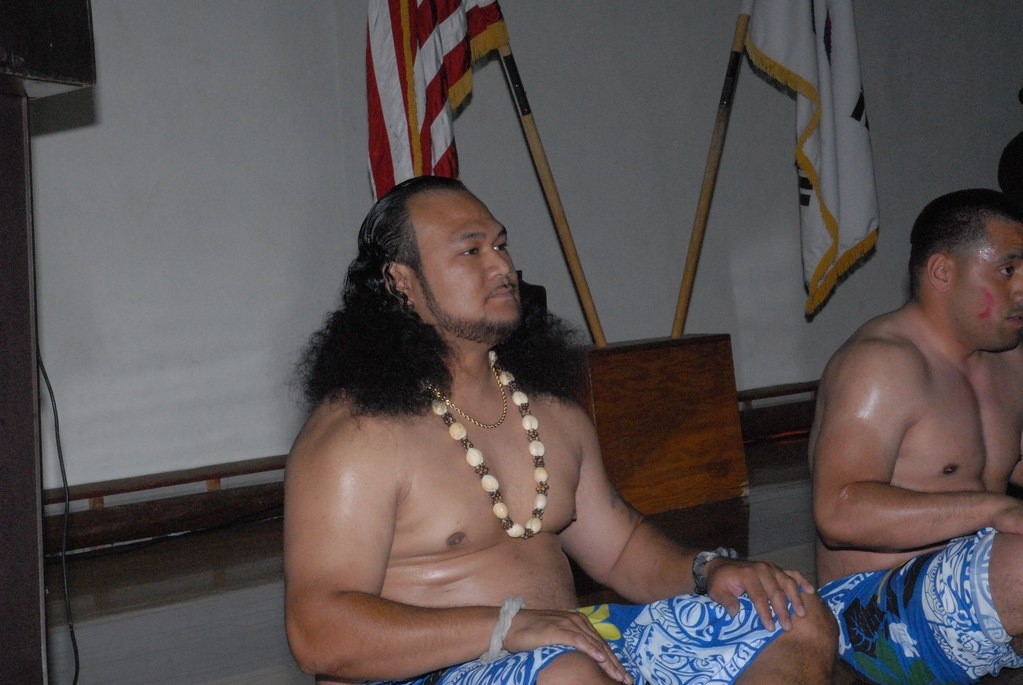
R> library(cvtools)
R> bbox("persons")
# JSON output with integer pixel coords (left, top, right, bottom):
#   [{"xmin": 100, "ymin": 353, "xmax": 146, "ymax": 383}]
[
  {"xmin": 284, "ymin": 175, "xmax": 840, "ymax": 685},
  {"xmin": 808, "ymin": 189, "xmax": 1023, "ymax": 685}
]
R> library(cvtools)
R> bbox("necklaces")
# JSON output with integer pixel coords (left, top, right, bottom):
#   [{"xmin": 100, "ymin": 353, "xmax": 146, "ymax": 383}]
[{"xmin": 420, "ymin": 349, "xmax": 549, "ymax": 540}]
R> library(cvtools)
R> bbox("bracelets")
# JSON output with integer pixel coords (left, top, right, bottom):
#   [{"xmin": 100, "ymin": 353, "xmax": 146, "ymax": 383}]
[
  {"xmin": 479, "ymin": 594, "xmax": 526, "ymax": 665},
  {"xmin": 692, "ymin": 546, "xmax": 738, "ymax": 595}
]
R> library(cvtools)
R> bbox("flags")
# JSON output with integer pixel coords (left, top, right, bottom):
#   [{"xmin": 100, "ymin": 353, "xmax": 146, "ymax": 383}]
[
  {"xmin": 363, "ymin": 0, "xmax": 510, "ymax": 201},
  {"xmin": 741, "ymin": 1, "xmax": 879, "ymax": 324}
]
